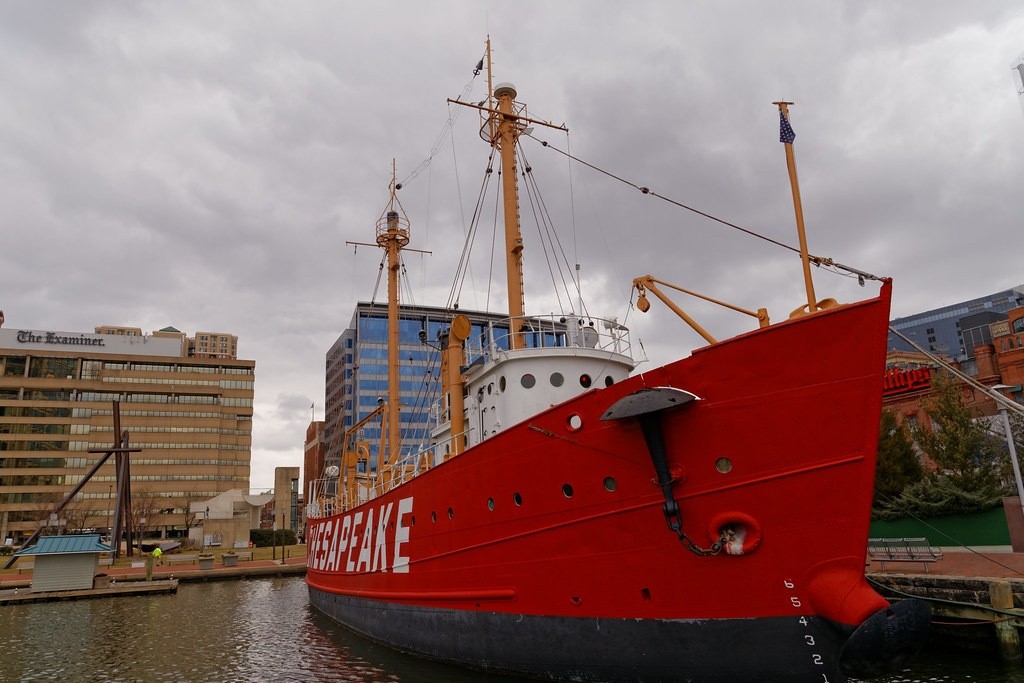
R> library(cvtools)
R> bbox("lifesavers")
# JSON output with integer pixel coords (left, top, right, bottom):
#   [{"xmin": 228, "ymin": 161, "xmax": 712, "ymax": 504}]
[{"xmin": 329, "ymin": 465, "xmax": 340, "ymax": 476}]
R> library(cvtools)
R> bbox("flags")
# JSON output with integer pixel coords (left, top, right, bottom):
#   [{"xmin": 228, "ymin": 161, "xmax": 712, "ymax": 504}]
[{"xmin": 778, "ymin": 111, "xmax": 797, "ymax": 143}]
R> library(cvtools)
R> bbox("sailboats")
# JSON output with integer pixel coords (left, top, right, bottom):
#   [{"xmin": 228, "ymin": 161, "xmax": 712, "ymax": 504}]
[{"xmin": 304, "ymin": 34, "xmax": 894, "ymax": 683}]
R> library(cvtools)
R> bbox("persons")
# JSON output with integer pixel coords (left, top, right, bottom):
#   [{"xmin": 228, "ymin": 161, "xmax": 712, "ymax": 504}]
[{"xmin": 152, "ymin": 545, "xmax": 163, "ymax": 567}]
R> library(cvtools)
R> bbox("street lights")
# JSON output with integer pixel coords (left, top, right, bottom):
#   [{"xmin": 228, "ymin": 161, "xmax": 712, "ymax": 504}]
[
  {"xmin": 281, "ymin": 508, "xmax": 288, "ymax": 565},
  {"xmin": 984, "ymin": 384, "xmax": 1024, "ymax": 515},
  {"xmin": 271, "ymin": 510, "xmax": 277, "ymax": 560}
]
